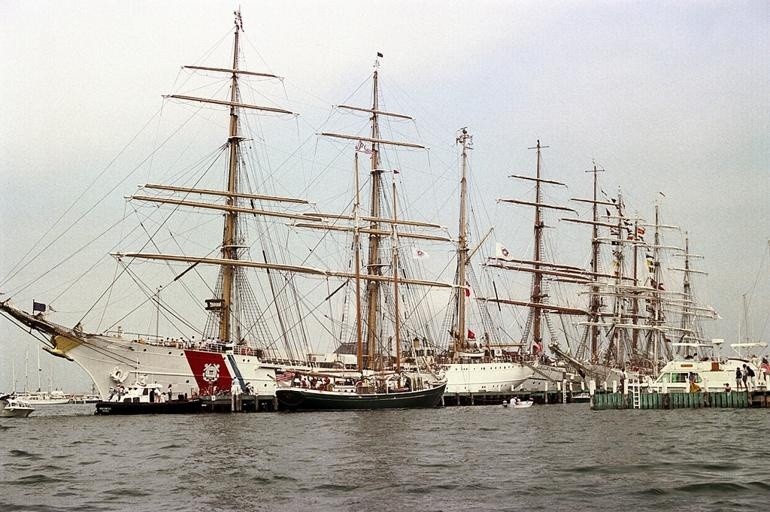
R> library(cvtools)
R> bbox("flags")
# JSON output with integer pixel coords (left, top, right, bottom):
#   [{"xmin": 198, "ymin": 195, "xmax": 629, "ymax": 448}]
[
  {"xmin": 496, "ymin": 242, "xmax": 512, "ymax": 261},
  {"xmin": 468, "ymin": 330, "xmax": 475, "ymax": 339},
  {"xmin": 33, "ymin": 302, "xmax": 46, "ymax": 312},
  {"xmin": 411, "ymin": 246, "xmax": 429, "ymax": 258}
]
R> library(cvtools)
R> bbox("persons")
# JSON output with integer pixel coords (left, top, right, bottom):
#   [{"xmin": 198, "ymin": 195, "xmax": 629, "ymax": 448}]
[
  {"xmin": 302, "ymin": 376, "xmax": 330, "ymax": 391},
  {"xmin": 159, "ymin": 336, "xmax": 237, "ymax": 352},
  {"xmin": 619, "ymin": 367, "xmax": 626, "ymax": 394},
  {"xmin": 685, "ymin": 353, "xmax": 770, "ymax": 393},
  {"xmin": 109, "ymin": 383, "xmax": 172, "ymax": 404},
  {"xmin": 209, "ymin": 377, "xmax": 254, "ymax": 396},
  {"xmin": 503, "ymin": 396, "xmax": 521, "ymax": 407}
]
